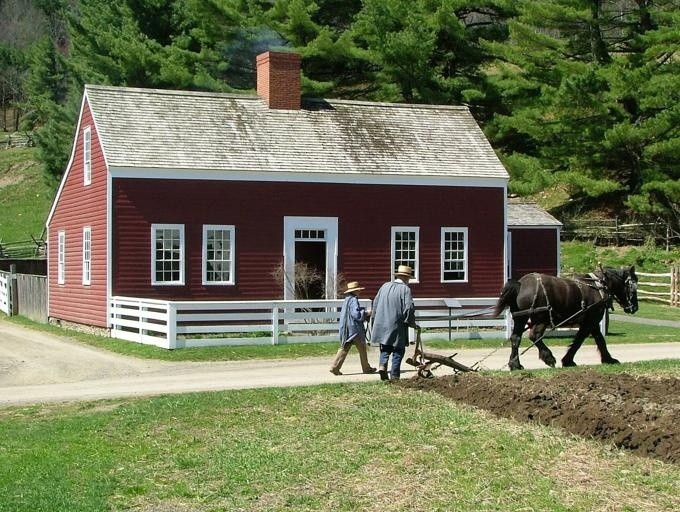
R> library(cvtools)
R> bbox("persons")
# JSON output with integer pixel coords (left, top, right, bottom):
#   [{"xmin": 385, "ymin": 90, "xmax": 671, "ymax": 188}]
[
  {"xmin": 366, "ymin": 265, "xmax": 416, "ymax": 381},
  {"xmin": 329, "ymin": 281, "xmax": 377, "ymax": 375}
]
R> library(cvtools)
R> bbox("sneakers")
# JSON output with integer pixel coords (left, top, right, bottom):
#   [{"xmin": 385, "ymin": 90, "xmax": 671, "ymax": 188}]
[{"xmin": 330, "ymin": 367, "xmax": 389, "ymax": 380}]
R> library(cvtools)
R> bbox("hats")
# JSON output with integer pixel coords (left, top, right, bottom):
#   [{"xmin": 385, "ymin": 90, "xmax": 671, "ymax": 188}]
[
  {"xmin": 344, "ymin": 282, "xmax": 365, "ymax": 293},
  {"xmin": 393, "ymin": 265, "xmax": 415, "ymax": 279}
]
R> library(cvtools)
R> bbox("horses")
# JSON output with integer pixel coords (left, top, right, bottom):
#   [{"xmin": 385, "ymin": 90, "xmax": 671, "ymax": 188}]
[{"xmin": 494, "ymin": 266, "xmax": 638, "ymax": 371}]
